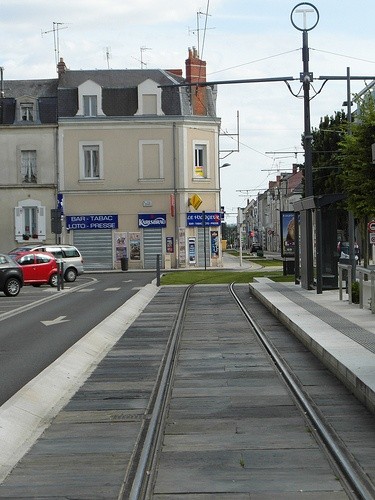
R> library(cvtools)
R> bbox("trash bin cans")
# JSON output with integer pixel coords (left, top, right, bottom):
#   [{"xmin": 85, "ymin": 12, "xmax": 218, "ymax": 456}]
[{"xmin": 120, "ymin": 255, "xmax": 128, "ymax": 271}]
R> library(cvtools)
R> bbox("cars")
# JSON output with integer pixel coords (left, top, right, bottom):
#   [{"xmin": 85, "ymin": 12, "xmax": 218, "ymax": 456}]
[
  {"xmin": 250, "ymin": 243, "xmax": 262, "ymax": 253},
  {"xmin": 0, "ymin": 253, "xmax": 24, "ymax": 296},
  {"xmin": 8, "ymin": 251, "xmax": 61, "ymax": 288}
]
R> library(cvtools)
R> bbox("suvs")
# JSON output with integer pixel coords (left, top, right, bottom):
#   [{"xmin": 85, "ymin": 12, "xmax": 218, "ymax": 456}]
[{"xmin": 11, "ymin": 243, "xmax": 84, "ymax": 283}]
